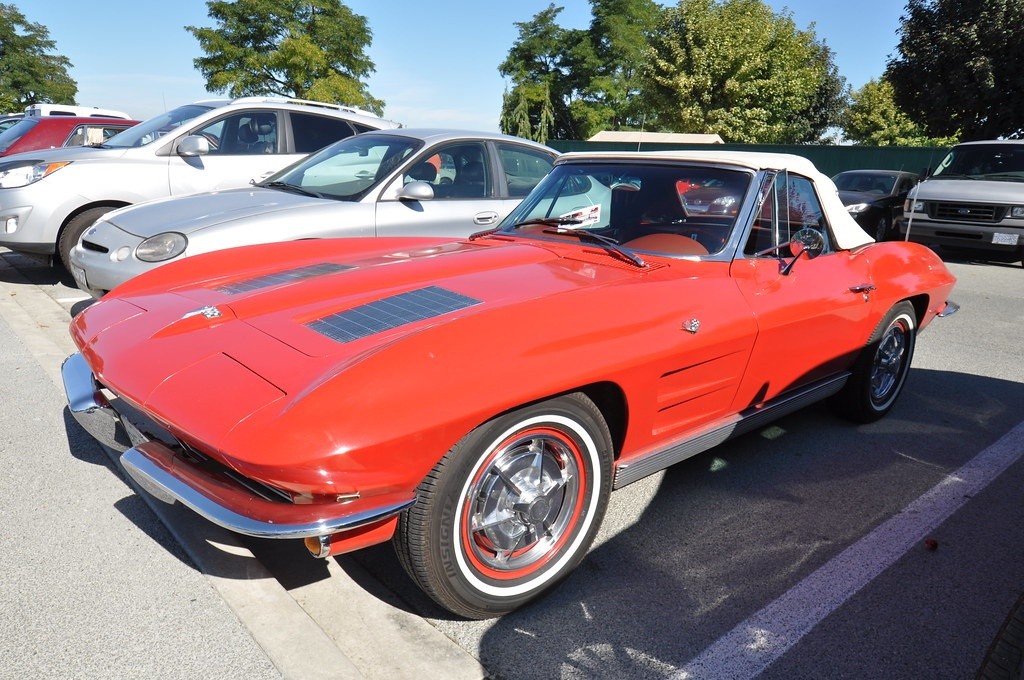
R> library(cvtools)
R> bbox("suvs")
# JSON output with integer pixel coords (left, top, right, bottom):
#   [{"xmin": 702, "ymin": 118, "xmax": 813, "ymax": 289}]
[
  {"xmin": 0, "ymin": 93, "xmax": 442, "ymax": 281},
  {"xmin": 900, "ymin": 139, "xmax": 1024, "ymax": 265}
]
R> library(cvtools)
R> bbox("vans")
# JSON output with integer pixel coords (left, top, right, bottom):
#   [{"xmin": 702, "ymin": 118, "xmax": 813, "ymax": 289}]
[{"xmin": 24, "ymin": 104, "xmax": 135, "ymax": 147}]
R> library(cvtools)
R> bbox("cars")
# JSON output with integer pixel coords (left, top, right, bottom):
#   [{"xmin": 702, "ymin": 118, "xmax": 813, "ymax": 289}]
[
  {"xmin": 0, "ymin": 116, "xmax": 221, "ymax": 159},
  {"xmin": 0, "ymin": 113, "xmax": 27, "ymax": 134},
  {"xmin": 59, "ymin": 148, "xmax": 963, "ymax": 623},
  {"xmin": 828, "ymin": 169, "xmax": 924, "ymax": 241},
  {"xmin": 68, "ymin": 127, "xmax": 613, "ymax": 304},
  {"xmin": 684, "ymin": 172, "xmax": 816, "ymax": 224}
]
